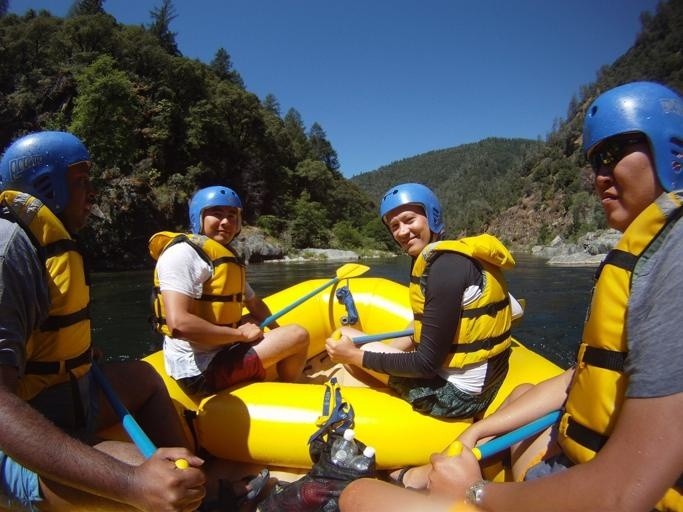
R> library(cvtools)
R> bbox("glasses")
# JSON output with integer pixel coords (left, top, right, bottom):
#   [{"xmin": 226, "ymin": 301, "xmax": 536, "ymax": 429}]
[{"xmin": 588, "ymin": 135, "xmax": 645, "ymax": 173}]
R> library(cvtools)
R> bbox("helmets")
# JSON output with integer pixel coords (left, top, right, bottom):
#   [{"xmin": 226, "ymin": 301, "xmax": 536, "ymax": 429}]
[
  {"xmin": 0, "ymin": 130, "xmax": 90, "ymax": 213},
  {"xmin": 380, "ymin": 183, "xmax": 444, "ymax": 233},
  {"xmin": 582, "ymin": 79, "xmax": 682, "ymax": 194},
  {"xmin": 189, "ymin": 185, "xmax": 243, "ymax": 233}
]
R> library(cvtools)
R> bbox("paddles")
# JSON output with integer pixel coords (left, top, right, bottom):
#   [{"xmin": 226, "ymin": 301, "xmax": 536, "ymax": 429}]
[
  {"xmin": 260, "ymin": 263, "xmax": 370, "ymax": 330},
  {"xmin": 331, "ymin": 299, "xmax": 527, "ymax": 346}
]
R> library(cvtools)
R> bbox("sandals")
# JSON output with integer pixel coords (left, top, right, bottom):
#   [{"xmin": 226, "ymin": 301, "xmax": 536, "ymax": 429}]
[
  {"xmin": 375, "ymin": 464, "xmax": 417, "ymax": 487},
  {"xmin": 198, "ymin": 468, "xmax": 270, "ymax": 511}
]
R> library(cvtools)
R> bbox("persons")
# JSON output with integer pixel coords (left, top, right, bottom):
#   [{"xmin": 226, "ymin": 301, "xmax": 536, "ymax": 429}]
[
  {"xmin": 0, "ymin": 127, "xmax": 275, "ymax": 512},
  {"xmin": 147, "ymin": 184, "xmax": 311, "ymax": 397},
  {"xmin": 322, "ymin": 180, "xmax": 519, "ymax": 423},
  {"xmin": 334, "ymin": 78, "xmax": 681, "ymax": 512}
]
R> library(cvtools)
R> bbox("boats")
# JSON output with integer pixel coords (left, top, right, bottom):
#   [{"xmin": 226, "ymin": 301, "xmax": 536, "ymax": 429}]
[{"xmin": 112, "ymin": 274, "xmax": 682, "ymax": 511}]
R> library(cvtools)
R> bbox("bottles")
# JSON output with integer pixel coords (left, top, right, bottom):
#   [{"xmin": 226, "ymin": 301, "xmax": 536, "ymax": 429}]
[
  {"xmin": 330, "ymin": 428, "xmax": 358, "ymax": 463},
  {"xmin": 265, "ymin": 479, "xmax": 334, "ymax": 512},
  {"xmin": 330, "ymin": 449, "xmax": 346, "ymax": 467},
  {"xmin": 347, "ymin": 445, "xmax": 375, "ymax": 471}
]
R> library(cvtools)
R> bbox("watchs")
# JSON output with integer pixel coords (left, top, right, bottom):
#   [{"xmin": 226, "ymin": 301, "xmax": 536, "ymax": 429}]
[{"xmin": 464, "ymin": 479, "xmax": 494, "ymax": 507}]
